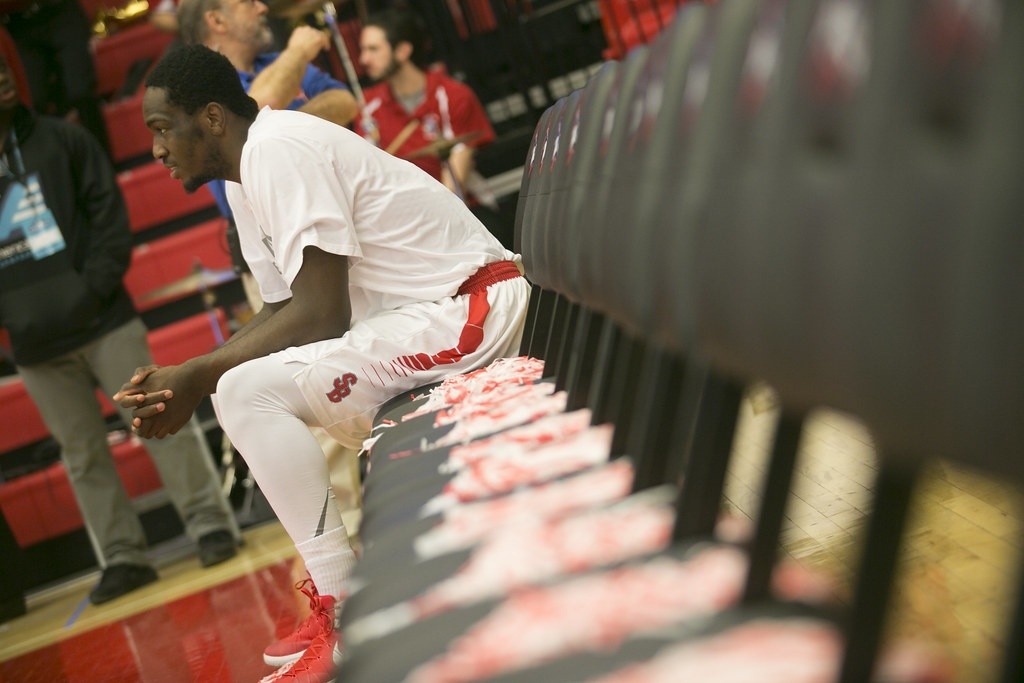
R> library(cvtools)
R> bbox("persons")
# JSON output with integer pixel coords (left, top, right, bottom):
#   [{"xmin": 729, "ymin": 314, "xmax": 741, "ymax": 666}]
[
  {"xmin": 349, "ymin": 20, "xmax": 498, "ymax": 234},
  {"xmin": 3, "ymin": 2, "xmax": 114, "ymax": 172},
  {"xmin": 0, "ymin": 55, "xmax": 240, "ymax": 607},
  {"xmin": 180, "ymin": 1, "xmax": 362, "ymax": 557},
  {"xmin": 113, "ymin": 42, "xmax": 531, "ymax": 678}
]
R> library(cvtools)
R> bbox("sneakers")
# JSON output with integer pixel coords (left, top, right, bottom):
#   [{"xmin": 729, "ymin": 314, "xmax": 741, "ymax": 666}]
[
  {"xmin": 261, "ymin": 587, "xmax": 349, "ymax": 683},
  {"xmin": 263, "ymin": 570, "xmax": 329, "ymax": 667}
]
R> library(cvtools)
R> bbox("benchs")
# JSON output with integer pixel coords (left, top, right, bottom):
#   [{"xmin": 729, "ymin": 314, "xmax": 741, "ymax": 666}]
[{"xmin": 0, "ymin": 0, "xmax": 250, "ymax": 548}]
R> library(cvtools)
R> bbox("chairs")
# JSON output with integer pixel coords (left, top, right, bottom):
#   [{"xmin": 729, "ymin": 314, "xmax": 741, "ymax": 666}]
[{"xmin": 330, "ymin": 2, "xmax": 1024, "ymax": 683}]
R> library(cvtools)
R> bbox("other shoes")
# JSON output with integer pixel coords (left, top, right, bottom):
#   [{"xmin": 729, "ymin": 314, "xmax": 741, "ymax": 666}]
[
  {"xmin": 196, "ymin": 528, "xmax": 241, "ymax": 567},
  {"xmin": 89, "ymin": 561, "xmax": 158, "ymax": 605},
  {"xmin": 0, "ymin": 598, "xmax": 26, "ymax": 625}
]
